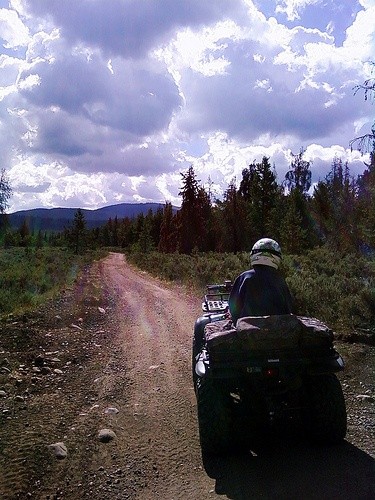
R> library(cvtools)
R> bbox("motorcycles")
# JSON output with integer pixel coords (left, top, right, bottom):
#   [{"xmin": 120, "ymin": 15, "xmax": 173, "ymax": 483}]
[{"xmin": 192, "ymin": 283, "xmax": 350, "ymax": 485}]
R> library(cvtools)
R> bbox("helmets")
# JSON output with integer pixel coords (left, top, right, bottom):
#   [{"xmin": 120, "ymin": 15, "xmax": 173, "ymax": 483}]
[{"xmin": 250, "ymin": 238, "xmax": 283, "ymax": 270}]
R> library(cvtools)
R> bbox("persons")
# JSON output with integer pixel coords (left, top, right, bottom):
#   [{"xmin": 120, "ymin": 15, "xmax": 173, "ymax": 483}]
[{"xmin": 228, "ymin": 238, "xmax": 296, "ymax": 326}]
